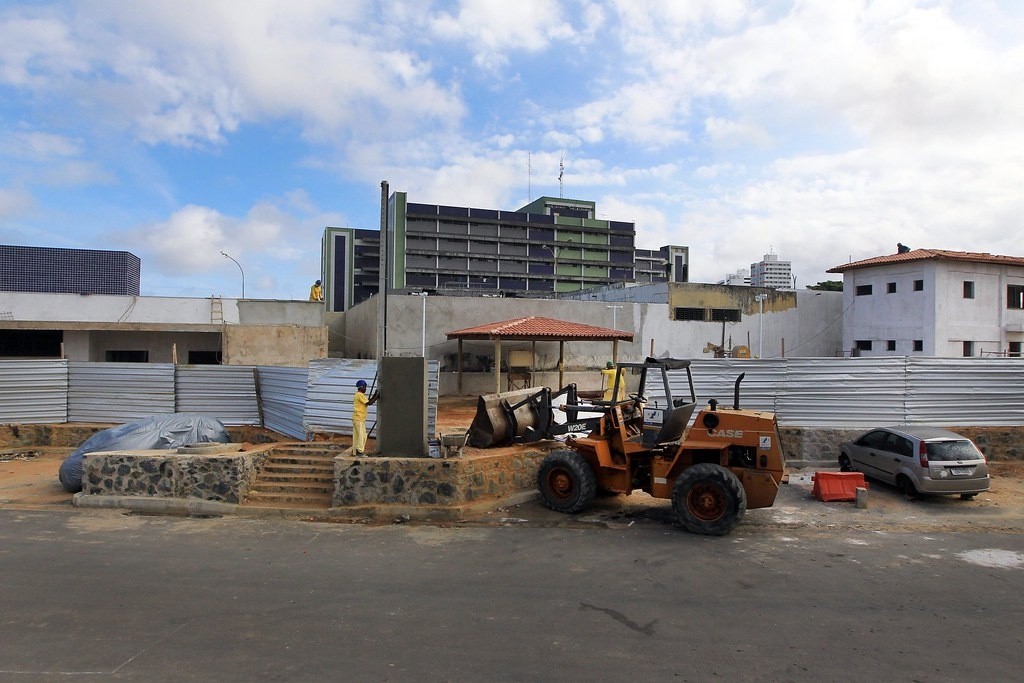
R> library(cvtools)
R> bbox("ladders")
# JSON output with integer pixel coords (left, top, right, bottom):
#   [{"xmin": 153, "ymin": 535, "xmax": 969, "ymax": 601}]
[{"xmin": 210, "ymin": 295, "xmax": 224, "ymax": 325}]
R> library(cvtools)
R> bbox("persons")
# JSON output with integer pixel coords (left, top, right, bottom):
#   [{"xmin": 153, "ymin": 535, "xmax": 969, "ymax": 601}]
[
  {"xmin": 351, "ymin": 380, "xmax": 380, "ymax": 458},
  {"xmin": 309, "ymin": 280, "xmax": 324, "ymax": 302},
  {"xmin": 616, "ymin": 367, "xmax": 627, "ymax": 401},
  {"xmin": 600, "ymin": 361, "xmax": 620, "ymax": 401}
]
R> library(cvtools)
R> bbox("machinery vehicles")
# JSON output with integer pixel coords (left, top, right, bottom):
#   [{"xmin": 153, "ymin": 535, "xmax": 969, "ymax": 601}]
[{"xmin": 464, "ymin": 357, "xmax": 790, "ymax": 536}]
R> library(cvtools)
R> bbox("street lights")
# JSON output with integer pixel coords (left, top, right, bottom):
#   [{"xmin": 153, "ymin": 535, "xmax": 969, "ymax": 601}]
[
  {"xmin": 542, "ymin": 238, "xmax": 573, "ymax": 300},
  {"xmin": 607, "ymin": 306, "xmax": 623, "ymax": 331},
  {"xmin": 220, "ymin": 251, "xmax": 244, "ymax": 299},
  {"xmin": 754, "ymin": 293, "xmax": 768, "ymax": 358}
]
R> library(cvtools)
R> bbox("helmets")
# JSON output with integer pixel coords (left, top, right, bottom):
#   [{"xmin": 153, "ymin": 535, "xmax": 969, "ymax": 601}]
[
  {"xmin": 606, "ymin": 361, "xmax": 614, "ymax": 368},
  {"xmin": 356, "ymin": 380, "xmax": 368, "ymax": 387},
  {"xmin": 316, "ymin": 280, "xmax": 321, "ymax": 285}
]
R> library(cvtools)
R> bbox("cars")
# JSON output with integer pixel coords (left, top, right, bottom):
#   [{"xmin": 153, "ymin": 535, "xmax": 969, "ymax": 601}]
[{"xmin": 837, "ymin": 425, "xmax": 991, "ymax": 502}]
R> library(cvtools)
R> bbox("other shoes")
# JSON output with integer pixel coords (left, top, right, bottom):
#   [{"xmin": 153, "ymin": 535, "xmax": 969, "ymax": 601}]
[{"xmin": 355, "ymin": 453, "xmax": 368, "ymax": 457}]
